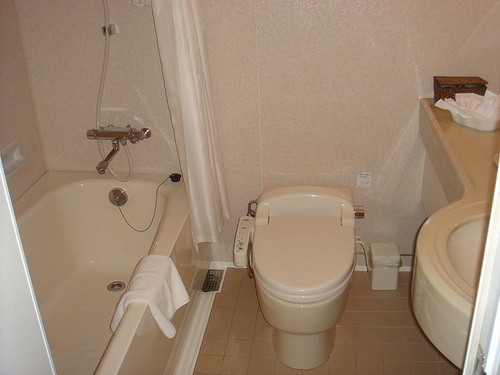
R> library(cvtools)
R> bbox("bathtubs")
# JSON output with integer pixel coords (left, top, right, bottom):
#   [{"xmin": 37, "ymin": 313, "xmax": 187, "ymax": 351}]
[{"xmin": 16, "ymin": 170, "xmax": 190, "ymax": 375}]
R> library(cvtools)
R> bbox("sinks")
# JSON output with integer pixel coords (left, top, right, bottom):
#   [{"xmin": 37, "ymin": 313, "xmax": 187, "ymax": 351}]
[{"xmin": 411, "ymin": 197, "xmax": 495, "ymax": 370}]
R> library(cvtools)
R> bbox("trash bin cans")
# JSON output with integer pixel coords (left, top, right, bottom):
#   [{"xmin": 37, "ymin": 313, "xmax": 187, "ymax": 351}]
[{"xmin": 369, "ymin": 242, "xmax": 401, "ymax": 290}]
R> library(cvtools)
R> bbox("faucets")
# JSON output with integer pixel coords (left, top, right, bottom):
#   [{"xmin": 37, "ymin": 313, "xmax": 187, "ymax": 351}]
[{"xmin": 94, "ymin": 138, "xmax": 120, "ymax": 173}]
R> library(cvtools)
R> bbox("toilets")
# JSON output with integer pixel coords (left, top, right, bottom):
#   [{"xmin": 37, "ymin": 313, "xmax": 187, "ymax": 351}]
[{"xmin": 233, "ymin": 185, "xmax": 363, "ymax": 369}]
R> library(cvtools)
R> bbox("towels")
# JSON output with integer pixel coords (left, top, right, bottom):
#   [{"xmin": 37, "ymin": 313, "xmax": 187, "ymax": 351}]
[{"xmin": 110, "ymin": 255, "xmax": 190, "ymax": 339}]
